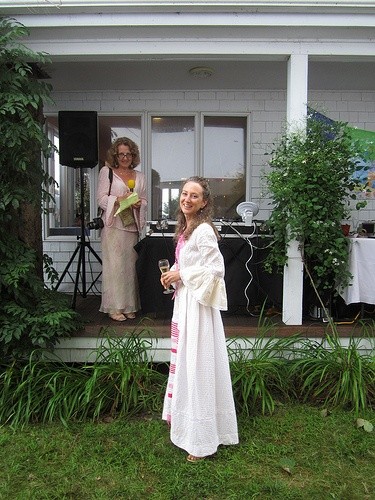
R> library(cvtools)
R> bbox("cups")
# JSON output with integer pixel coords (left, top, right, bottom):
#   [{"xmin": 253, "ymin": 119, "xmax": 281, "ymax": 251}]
[{"xmin": 342, "ymin": 225, "xmax": 350, "ymax": 237}]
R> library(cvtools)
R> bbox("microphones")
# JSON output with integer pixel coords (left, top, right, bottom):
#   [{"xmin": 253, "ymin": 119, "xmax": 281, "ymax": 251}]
[{"xmin": 128, "ymin": 179, "xmax": 135, "ymax": 194}]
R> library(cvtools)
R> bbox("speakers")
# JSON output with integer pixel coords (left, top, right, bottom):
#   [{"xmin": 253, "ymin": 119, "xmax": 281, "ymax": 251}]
[{"xmin": 58, "ymin": 111, "xmax": 98, "ymax": 169}]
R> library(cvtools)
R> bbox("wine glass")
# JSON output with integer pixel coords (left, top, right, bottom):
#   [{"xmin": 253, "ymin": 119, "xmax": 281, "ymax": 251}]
[{"xmin": 159, "ymin": 258, "xmax": 175, "ymax": 295}]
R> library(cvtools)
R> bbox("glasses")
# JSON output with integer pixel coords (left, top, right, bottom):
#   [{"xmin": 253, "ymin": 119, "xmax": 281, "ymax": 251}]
[{"xmin": 118, "ymin": 153, "xmax": 132, "ymax": 158}]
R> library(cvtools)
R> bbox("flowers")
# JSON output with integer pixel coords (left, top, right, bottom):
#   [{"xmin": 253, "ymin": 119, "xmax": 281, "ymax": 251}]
[{"xmin": 258, "ymin": 94, "xmax": 371, "ymax": 288}]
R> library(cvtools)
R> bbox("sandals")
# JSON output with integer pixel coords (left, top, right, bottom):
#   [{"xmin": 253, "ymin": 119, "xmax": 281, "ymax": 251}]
[
  {"xmin": 125, "ymin": 312, "xmax": 135, "ymax": 318},
  {"xmin": 108, "ymin": 313, "xmax": 126, "ymax": 321}
]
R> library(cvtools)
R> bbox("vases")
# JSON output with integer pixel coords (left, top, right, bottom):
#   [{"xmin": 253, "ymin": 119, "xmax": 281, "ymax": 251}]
[{"xmin": 309, "ymin": 289, "xmax": 332, "ymax": 321}]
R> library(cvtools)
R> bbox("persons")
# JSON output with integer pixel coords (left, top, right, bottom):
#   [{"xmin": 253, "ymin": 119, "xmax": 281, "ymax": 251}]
[
  {"xmin": 151, "ymin": 169, "xmax": 163, "ymax": 221},
  {"xmin": 160, "ymin": 176, "xmax": 239, "ymax": 463},
  {"xmin": 98, "ymin": 137, "xmax": 148, "ymax": 321}
]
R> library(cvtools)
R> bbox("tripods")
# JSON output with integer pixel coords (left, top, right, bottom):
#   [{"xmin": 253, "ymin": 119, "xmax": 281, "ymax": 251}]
[{"xmin": 55, "ymin": 168, "xmax": 103, "ymax": 311}]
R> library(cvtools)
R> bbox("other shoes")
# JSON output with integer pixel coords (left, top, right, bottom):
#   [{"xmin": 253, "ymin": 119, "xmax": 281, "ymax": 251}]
[{"xmin": 187, "ymin": 454, "xmax": 207, "ymax": 463}]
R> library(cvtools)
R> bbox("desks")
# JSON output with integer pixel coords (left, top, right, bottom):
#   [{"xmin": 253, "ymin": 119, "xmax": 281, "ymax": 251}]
[
  {"xmin": 334, "ymin": 236, "xmax": 375, "ymax": 312},
  {"xmin": 133, "ymin": 232, "xmax": 284, "ymax": 315}
]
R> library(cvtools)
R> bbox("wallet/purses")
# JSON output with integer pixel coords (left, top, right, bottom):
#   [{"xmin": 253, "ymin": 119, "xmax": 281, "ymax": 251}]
[{"xmin": 119, "ymin": 209, "xmax": 135, "ymax": 227}]
